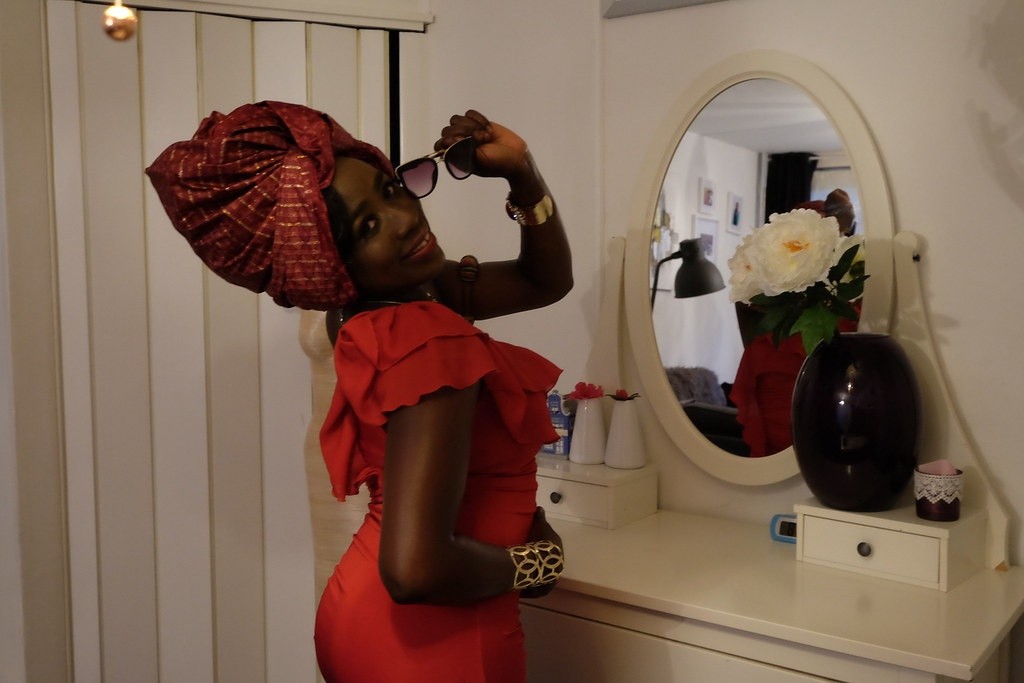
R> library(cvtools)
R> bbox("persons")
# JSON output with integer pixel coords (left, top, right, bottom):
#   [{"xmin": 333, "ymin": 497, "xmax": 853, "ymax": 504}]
[
  {"xmin": 728, "ymin": 188, "xmax": 858, "ymax": 457},
  {"xmin": 149, "ymin": 100, "xmax": 578, "ymax": 683}
]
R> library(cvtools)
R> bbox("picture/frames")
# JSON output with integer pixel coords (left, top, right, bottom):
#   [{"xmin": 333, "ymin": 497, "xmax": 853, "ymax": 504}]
[
  {"xmin": 690, "ymin": 213, "xmax": 720, "ymax": 269},
  {"xmin": 697, "ymin": 175, "xmax": 718, "ymax": 216},
  {"xmin": 725, "ymin": 190, "xmax": 748, "ymax": 237}
]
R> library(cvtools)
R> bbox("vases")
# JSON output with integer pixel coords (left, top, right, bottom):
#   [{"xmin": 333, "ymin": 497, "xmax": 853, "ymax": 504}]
[
  {"xmin": 787, "ymin": 330, "xmax": 926, "ymax": 516},
  {"xmin": 568, "ymin": 397, "xmax": 608, "ymax": 465},
  {"xmin": 604, "ymin": 399, "xmax": 647, "ymax": 470}
]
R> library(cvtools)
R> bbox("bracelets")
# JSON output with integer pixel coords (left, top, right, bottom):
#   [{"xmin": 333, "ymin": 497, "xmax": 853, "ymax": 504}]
[
  {"xmin": 506, "ymin": 185, "xmax": 556, "ymax": 226},
  {"xmin": 504, "ymin": 541, "xmax": 564, "ymax": 590}
]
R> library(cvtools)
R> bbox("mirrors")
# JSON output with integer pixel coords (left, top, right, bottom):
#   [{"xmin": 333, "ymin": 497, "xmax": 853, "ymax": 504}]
[{"xmin": 621, "ymin": 48, "xmax": 902, "ymax": 490}]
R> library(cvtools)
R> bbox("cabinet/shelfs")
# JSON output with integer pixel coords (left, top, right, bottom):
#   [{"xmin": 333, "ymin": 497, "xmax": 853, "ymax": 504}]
[{"xmin": 515, "ymin": 453, "xmax": 1023, "ymax": 683}]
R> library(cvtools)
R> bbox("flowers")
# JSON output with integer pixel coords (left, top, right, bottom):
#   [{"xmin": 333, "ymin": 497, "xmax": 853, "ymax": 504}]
[
  {"xmin": 604, "ymin": 387, "xmax": 642, "ymax": 401},
  {"xmin": 562, "ymin": 381, "xmax": 608, "ymax": 401},
  {"xmin": 726, "ymin": 205, "xmax": 875, "ymax": 359}
]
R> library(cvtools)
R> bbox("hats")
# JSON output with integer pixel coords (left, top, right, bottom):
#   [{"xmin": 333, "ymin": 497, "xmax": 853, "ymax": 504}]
[{"xmin": 143, "ymin": 98, "xmax": 399, "ymax": 312}]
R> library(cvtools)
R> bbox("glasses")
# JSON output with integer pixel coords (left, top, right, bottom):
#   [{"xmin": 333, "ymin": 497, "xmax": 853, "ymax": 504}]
[{"xmin": 395, "ymin": 133, "xmax": 477, "ymax": 200}]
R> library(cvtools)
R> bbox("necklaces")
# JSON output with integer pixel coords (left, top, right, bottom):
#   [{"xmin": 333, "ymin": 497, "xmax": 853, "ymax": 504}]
[{"xmin": 354, "ymin": 287, "xmax": 440, "ymax": 305}]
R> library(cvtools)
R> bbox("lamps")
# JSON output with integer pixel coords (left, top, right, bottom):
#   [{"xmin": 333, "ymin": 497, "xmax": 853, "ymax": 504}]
[
  {"xmin": 646, "ymin": 236, "xmax": 727, "ymax": 311},
  {"xmin": 101, "ymin": 0, "xmax": 140, "ymax": 41}
]
